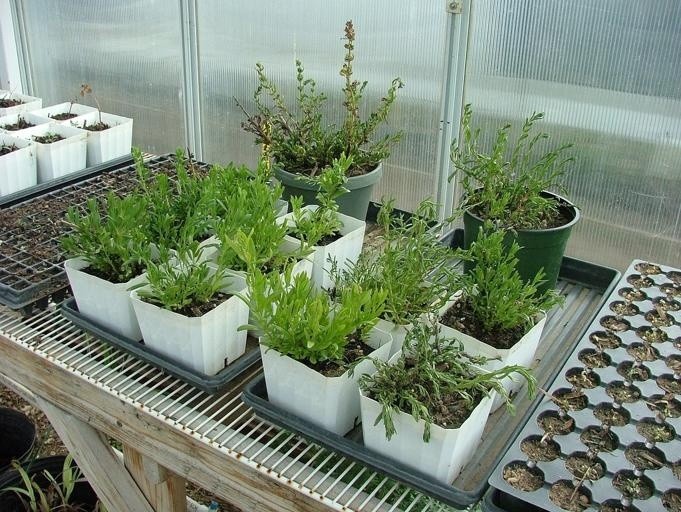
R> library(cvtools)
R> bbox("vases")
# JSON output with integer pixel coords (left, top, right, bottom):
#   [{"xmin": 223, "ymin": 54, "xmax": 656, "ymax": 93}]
[{"xmin": 0, "ymin": 90, "xmax": 133, "ymax": 195}]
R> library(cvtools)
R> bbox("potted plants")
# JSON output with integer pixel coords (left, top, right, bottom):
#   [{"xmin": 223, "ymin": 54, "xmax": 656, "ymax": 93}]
[
  {"xmin": 357, "ymin": 311, "xmax": 537, "ymax": 484},
  {"xmin": 329, "ymin": 194, "xmax": 457, "ymax": 356},
  {"xmin": 0, "ymin": 452, "xmax": 100, "ymax": 512},
  {"xmin": 420, "ymin": 224, "xmax": 546, "ymax": 414},
  {"xmin": 232, "ymin": 19, "xmax": 407, "ymax": 222},
  {"xmin": 442, "ymin": 102, "xmax": 582, "ymax": 306}
]
